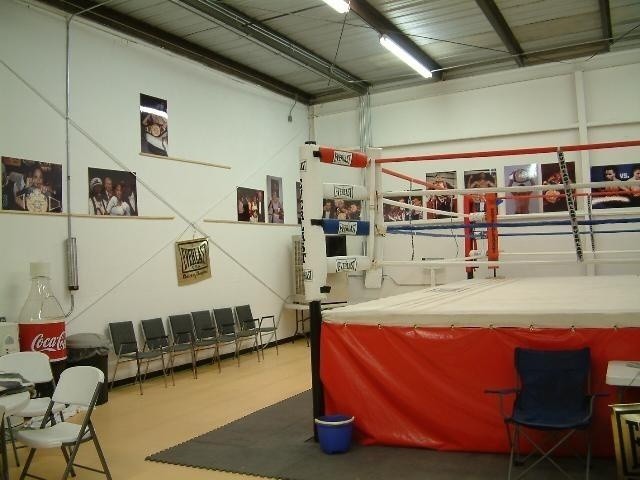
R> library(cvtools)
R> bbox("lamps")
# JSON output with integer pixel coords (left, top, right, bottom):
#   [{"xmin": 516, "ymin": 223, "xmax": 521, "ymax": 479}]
[
  {"xmin": 377, "ymin": 33, "xmax": 432, "ymax": 80},
  {"xmin": 323, "ymin": 0, "xmax": 351, "ymax": 14}
]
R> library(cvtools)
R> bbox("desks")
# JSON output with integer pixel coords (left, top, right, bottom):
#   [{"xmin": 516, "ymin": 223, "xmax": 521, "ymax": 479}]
[
  {"xmin": 0, "ymin": 370, "xmax": 31, "ymax": 480},
  {"xmin": 285, "ymin": 301, "xmax": 349, "ymax": 347},
  {"xmin": 605, "ymin": 360, "xmax": 639, "ymax": 404}
]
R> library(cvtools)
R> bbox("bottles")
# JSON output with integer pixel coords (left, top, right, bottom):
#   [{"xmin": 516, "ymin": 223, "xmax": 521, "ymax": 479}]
[{"xmin": 16, "ymin": 260, "xmax": 68, "ymax": 388}]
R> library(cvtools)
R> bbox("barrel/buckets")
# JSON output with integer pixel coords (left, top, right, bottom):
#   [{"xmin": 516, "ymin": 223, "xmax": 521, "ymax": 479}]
[{"xmin": 313, "ymin": 413, "xmax": 355, "ymax": 455}]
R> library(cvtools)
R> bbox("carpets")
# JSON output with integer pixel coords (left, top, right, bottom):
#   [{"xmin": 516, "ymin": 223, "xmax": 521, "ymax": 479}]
[{"xmin": 143, "ymin": 387, "xmax": 617, "ymax": 479}]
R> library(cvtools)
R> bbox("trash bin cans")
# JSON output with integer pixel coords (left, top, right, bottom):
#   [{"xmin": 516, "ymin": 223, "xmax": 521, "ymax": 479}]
[{"xmin": 66, "ymin": 333, "xmax": 109, "ymax": 406}]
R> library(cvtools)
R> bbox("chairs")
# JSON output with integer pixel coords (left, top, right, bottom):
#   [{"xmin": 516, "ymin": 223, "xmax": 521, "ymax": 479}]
[
  {"xmin": 108, "ymin": 303, "xmax": 278, "ymax": 395},
  {"xmin": 0, "ymin": 351, "xmax": 74, "ymax": 466},
  {"xmin": 18, "ymin": 365, "xmax": 112, "ymax": 480},
  {"xmin": 483, "ymin": 346, "xmax": 611, "ymax": 480}
]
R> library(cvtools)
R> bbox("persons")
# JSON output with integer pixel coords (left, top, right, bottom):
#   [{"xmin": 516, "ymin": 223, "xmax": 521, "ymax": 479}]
[
  {"xmin": 145, "ymin": 100, "xmax": 168, "ymax": 154},
  {"xmin": 543, "ymin": 174, "xmax": 566, "ymax": 212},
  {"xmin": 599, "ymin": 168, "xmax": 640, "ymax": 203},
  {"xmin": 468, "ymin": 172, "xmax": 497, "ymax": 212},
  {"xmin": 388, "ymin": 198, "xmax": 423, "ymax": 220},
  {"xmin": 508, "ymin": 171, "xmax": 535, "ymax": 214},
  {"xmin": 1, "ymin": 156, "xmax": 131, "ymax": 217},
  {"xmin": 239, "ymin": 191, "xmax": 283, "ymax": 223},
  {"xmin": 323, "ymin": 198, "xmax": 359, "ymax": 221}
]
